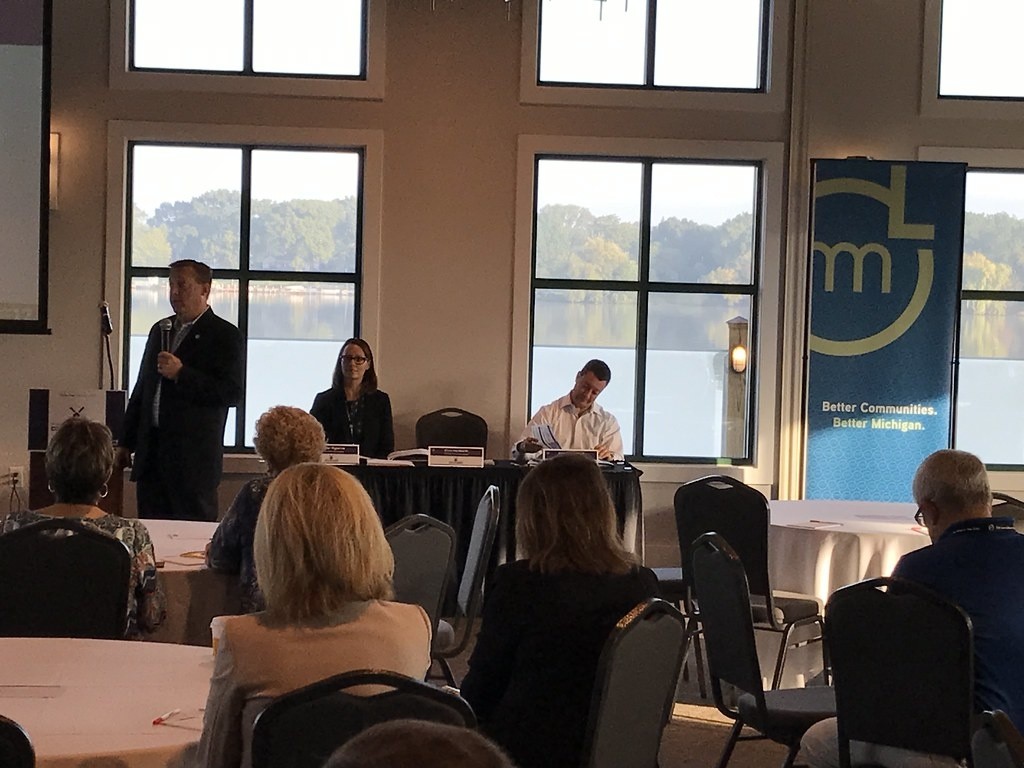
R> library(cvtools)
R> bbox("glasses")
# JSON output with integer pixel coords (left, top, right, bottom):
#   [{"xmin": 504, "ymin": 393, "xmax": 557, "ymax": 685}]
[
  {"xmin": 914, "ymin": 504, "xmax": 927, "ymax": 528},
  {"xmin": 338, "ymin": 354, "xmax": 368, "ymax": 365}
]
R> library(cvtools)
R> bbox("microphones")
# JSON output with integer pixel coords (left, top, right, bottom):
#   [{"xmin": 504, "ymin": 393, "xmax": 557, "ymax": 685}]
[
  {"xmin": 98, "ymin": 300, "xmax": 113, "ymax": 335},
  {"xmin": 159, "ymin": 319, "xmax": 172, "ymax": 354}
]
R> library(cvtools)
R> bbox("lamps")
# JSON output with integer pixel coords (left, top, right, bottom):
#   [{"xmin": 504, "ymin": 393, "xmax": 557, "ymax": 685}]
[{"xmin": 49, "ymin": 132, "xmax": 59, "ymax": 208}]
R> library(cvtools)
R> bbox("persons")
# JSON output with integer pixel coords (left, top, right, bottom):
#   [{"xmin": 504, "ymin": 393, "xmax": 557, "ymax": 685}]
[
  {"xmin": 193, "ymin": 462, "xmax": 432, "ymax": 768},
  {"xmin": 119, "ymin": 260, "xmax": 246, "ymax": 523},
  {"xmin": 791, "ymin": 450, "xmax": 1024, "ymax": 768},
  {"xmin": 0, "ymin": 416, "xmax": 167, "ymax": 642},
  {"xmin": 204, "ymin": 406, "xmax": 328, "ymax": 615},
  {"xmin": 310, "ymin": 337, "xmax": 396, "ymax": 461},
  {"xmin": 511, "ymin": 359, "xmax": 625, "ymax": 466},
  {"xmin": 323, "ymin": 720, "xmax": 515, "ymax": 768},
  {"xmin": 457, "ymin": 452, "xmax": 660, "ymax": 768}
]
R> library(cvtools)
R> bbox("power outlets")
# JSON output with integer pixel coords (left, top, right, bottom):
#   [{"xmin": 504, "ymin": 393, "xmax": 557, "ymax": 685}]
[{"xmin": 9, "ymin": 467, "xmax": 23, "ymax": 487}]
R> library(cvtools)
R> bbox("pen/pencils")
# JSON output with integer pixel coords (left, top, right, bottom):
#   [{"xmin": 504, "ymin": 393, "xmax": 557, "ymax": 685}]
[
  {"xmin": 152, "ymin": 709, "xmax": 180, "ymax": 724},
  {"xmin": 810, "ymin": 520, "xmax": 842, "ymax": 524}
]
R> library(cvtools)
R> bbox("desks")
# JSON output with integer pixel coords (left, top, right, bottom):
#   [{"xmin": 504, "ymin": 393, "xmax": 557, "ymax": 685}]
[
  {"xmin": 749, "ymin": 498, "xmax": 933, "ymax": 692},
  {"xmin": 0, "ymin": 638, "xmax": 215, "ymax": 768},
  {"xmin": 332, "ymin": 460, "xmax": 644, "ymax": 618},
  {"xmin": 131, "ymin": 519, "xmax": 246, "ymax": 648}
]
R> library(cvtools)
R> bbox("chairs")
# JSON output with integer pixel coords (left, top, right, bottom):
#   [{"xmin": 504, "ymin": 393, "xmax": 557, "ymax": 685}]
[{"xmin": 0, "ymin": 408, "xmax": 1024, "ymax": 768}]
